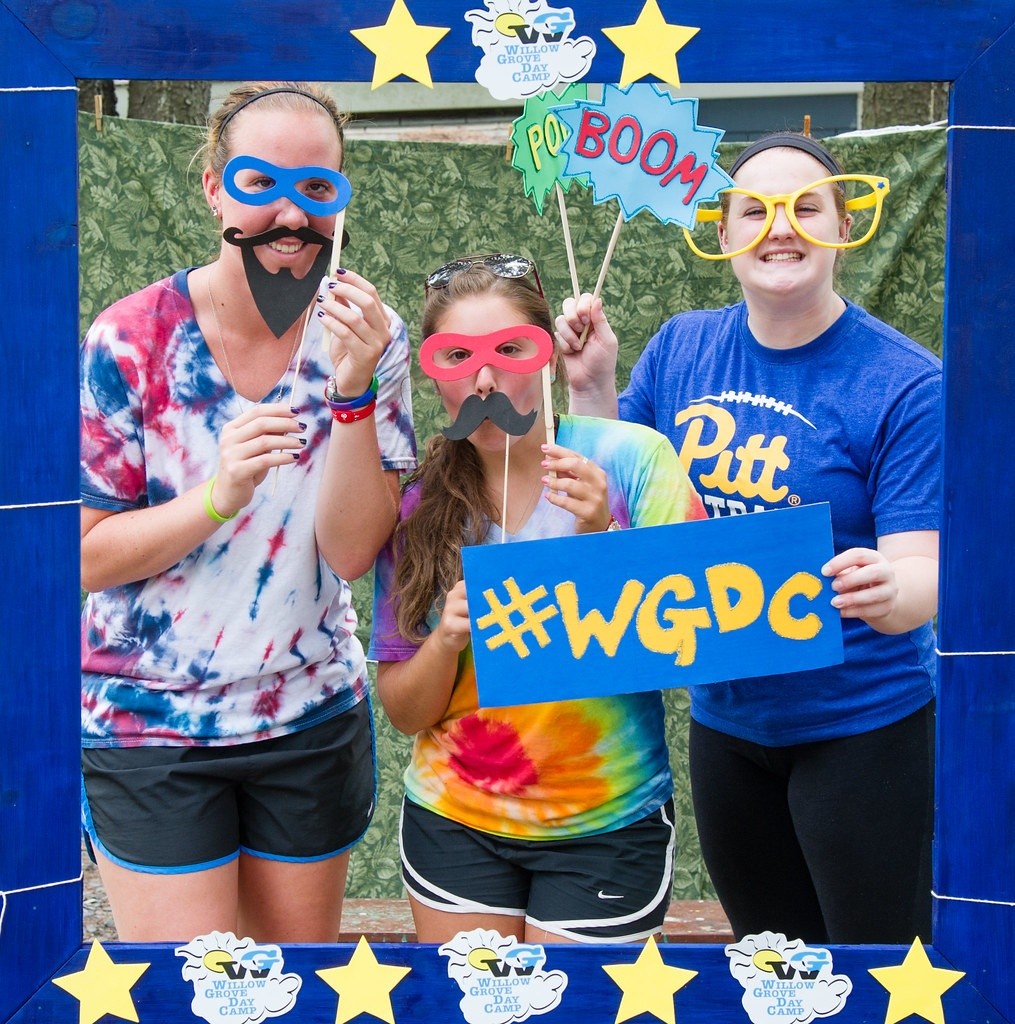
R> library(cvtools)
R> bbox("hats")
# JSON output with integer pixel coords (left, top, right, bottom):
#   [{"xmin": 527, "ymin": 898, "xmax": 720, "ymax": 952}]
[{"xmin": 727, "ymin": 133, "xmax": 846, "ymax": 199}]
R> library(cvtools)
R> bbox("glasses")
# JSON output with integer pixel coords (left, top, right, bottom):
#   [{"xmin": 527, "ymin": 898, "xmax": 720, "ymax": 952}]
[
  {"xmin": 418, "ymin": 325, "xmax": 552, "ymax": 382},
  {"xmin": 681, "ymin": 172, "xmax": 890, "ymax": 260},
  {"xmin": 425, "ymin": 254, "xmax": 544, "ymax": 311},
  {"xmin": 222, "ymin": 154, "xmax": 352, "ymax": 217}
]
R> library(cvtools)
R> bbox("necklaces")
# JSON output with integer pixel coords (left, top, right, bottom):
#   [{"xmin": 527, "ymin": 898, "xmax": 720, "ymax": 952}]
[
  {"xmin": 208, "ymin": 267, "xmax": 305, "ymax": 486},
  {"xmin": 503, "ymin": 480, "xmax": 538, "ymax": 535}
]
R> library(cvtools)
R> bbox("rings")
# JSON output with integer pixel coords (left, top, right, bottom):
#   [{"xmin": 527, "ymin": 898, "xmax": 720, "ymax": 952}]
[{"xmin": 583, "ymin": 458, "xmax": 588, "ymax": 463}]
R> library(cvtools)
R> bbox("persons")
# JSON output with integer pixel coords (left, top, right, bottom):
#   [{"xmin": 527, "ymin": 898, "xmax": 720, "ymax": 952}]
[
  {"xmin": 554, "ymin": 133, "xmax": 943, "ymax": 944},
  {"xmin": 369, "ymin": 264, "xmax": 708, "ymax": 945},
  {"xmin": 81, "ymin": 81, "xmax": 417, "ymax": 943}
]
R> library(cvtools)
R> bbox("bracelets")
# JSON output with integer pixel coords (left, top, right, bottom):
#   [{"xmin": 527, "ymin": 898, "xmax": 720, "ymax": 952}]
[
  {"xmin": 323, "ymin": 373, "xmax": 377, "ymax": 407},
  {"xmin": 607, "ymin": 516, "xmax": 621, "ymax": 531},
  {"xmin": 330, "ymin": 401, "xmax": 376, "ymax": 422},
  {"xmin": 326, "ymin": 377, "xmax": 357, "ymax": 402},
  {"xmin": 204, "ymin": 476, "xmax": 240, "ymax": 523}
]
